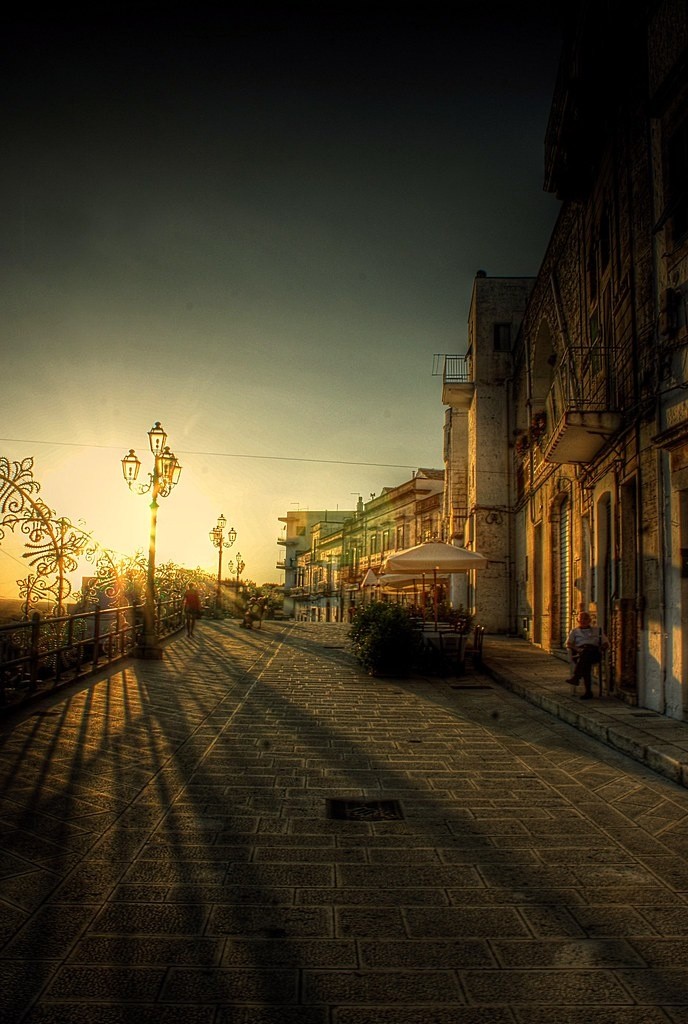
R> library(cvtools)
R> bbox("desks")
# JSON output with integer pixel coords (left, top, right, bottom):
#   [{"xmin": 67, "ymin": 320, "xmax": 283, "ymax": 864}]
[{"xmin": 411, "ymin": 617, "xmax": 467, "ymax": 679}]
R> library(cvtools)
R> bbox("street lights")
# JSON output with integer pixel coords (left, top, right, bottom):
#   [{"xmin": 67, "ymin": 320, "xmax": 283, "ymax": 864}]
[
  {"xmin": 210, "ymin": 514, "xmax": 236, "ymax": 618},
  {"xmin": 121, "ymin": 418, "xmax": 180, "ymax": 658},
  {"xmin": 228, "ymin": 552, "xmax": 245, "ymax": 618}
]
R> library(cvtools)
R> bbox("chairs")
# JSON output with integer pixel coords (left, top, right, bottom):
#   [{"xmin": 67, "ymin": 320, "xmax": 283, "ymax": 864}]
[{"xmin": 467, "ymin": 625, "xmax": 484, "ymax": 675}]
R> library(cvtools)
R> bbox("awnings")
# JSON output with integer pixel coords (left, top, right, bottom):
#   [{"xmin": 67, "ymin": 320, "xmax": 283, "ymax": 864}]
[{"xmin": 361, "ymin": 538, "xmax": 488, "ymax": 632}]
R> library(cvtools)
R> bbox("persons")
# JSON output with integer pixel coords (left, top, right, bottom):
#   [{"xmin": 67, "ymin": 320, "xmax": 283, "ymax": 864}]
[
  {"xmin": 564, "ymin": 612, "xmax": 610, "ymax": 698},
  {"xmin": 181, "ymin": 583, "xmax": 201, "ymax": 638}
]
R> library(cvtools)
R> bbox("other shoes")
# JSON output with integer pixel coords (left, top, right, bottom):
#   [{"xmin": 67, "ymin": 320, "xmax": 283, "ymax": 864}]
[
  {"xmin": 566, "ymin": 676, "xmax": 581, "ymax": 686},
  {"xmin": 579, "ymin": 691, "xmax": 593, "ymax": 700}
]
